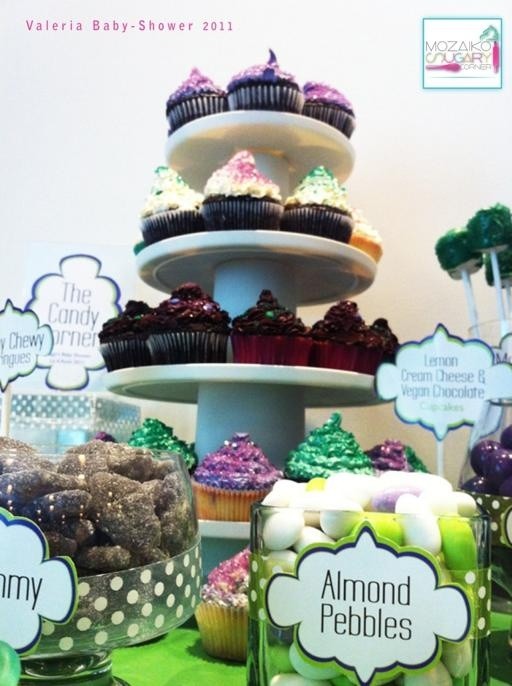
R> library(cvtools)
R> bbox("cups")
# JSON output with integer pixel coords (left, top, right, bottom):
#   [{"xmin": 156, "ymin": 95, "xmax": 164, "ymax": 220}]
[{"xmin": 243, "ymin": 497, "xmax": 493, "ymax": 686}]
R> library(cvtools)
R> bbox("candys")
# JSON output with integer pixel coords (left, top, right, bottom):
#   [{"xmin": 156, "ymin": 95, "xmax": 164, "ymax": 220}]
[
  {"xmin": 261, "ymin": 423, "xmax": 512, "ymax": 686},
  {"xmin": 433, "ymin": 201, "xmax": 512, "ymax": 290}
]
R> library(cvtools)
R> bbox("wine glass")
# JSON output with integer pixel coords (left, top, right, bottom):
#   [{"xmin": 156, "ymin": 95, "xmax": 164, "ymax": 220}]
[
  {"xmin": 0, "ymin": 441, "xmax": 206, "ymax": 686},
  {"xmin": 452, "ymin": 396, "xmax": 510, "ymax": 686}
]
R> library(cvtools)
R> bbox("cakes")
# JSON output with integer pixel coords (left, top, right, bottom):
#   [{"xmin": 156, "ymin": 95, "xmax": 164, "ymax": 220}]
[
  {"xmin": 93, "ymin": 47, "xmax": 430, "ymax": 522},
  {"xmin": 194, "ymin": 544, "xmax": 250, "ymax": 662}
]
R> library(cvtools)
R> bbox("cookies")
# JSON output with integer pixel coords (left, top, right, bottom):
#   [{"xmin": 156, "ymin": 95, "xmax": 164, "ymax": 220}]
[{"xmin": 0, "ymin": 434, "xmax": 198, "ymax": 579}]
